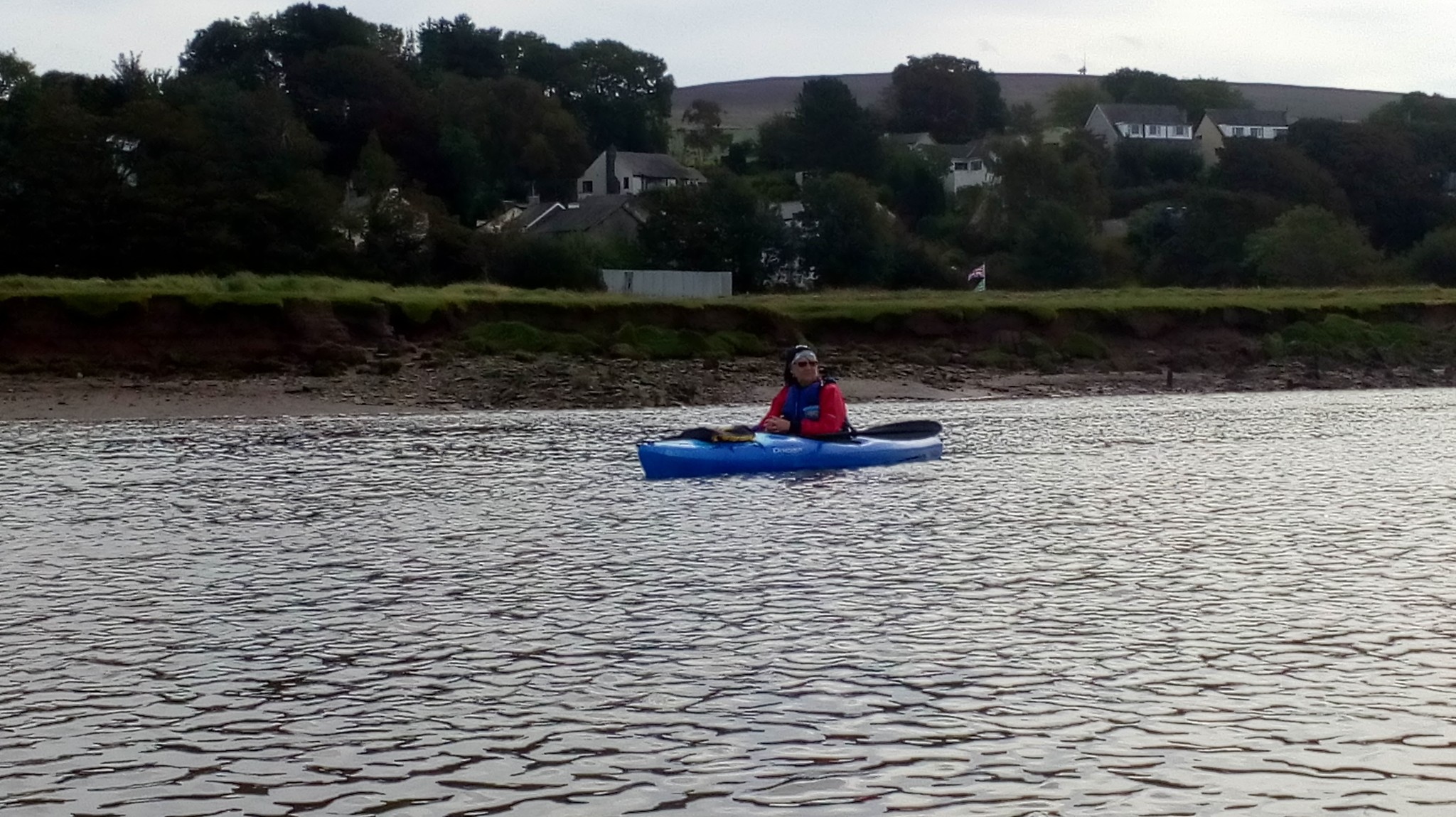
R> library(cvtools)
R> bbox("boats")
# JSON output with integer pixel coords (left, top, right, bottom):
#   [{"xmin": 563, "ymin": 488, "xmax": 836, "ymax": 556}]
[{"xmin": 638, "ymin": 415, "xmax": 945, "ymax": 482}]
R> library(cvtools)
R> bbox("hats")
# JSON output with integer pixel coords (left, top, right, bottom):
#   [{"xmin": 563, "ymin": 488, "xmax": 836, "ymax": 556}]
[{"xmin": 784, "ymin": 345, "xmax": 811, "ymax": 385}]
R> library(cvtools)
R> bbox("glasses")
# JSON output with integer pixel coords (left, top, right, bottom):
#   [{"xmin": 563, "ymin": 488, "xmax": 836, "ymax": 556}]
[{"xmin": 794, "ymin": 362, "xmax": 818, "ymax": 367}]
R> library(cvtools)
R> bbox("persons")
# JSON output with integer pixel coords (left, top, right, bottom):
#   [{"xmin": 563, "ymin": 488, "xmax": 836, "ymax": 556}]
[{"xmin": 760, "ymin": 345, "xmax": 851, "ymax": 436}]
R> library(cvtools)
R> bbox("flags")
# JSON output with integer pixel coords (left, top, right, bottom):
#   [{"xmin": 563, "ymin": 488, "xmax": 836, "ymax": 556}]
[{"xmin": 968, "ymin": 264, "xmax": 985, "ymax": 280}]
